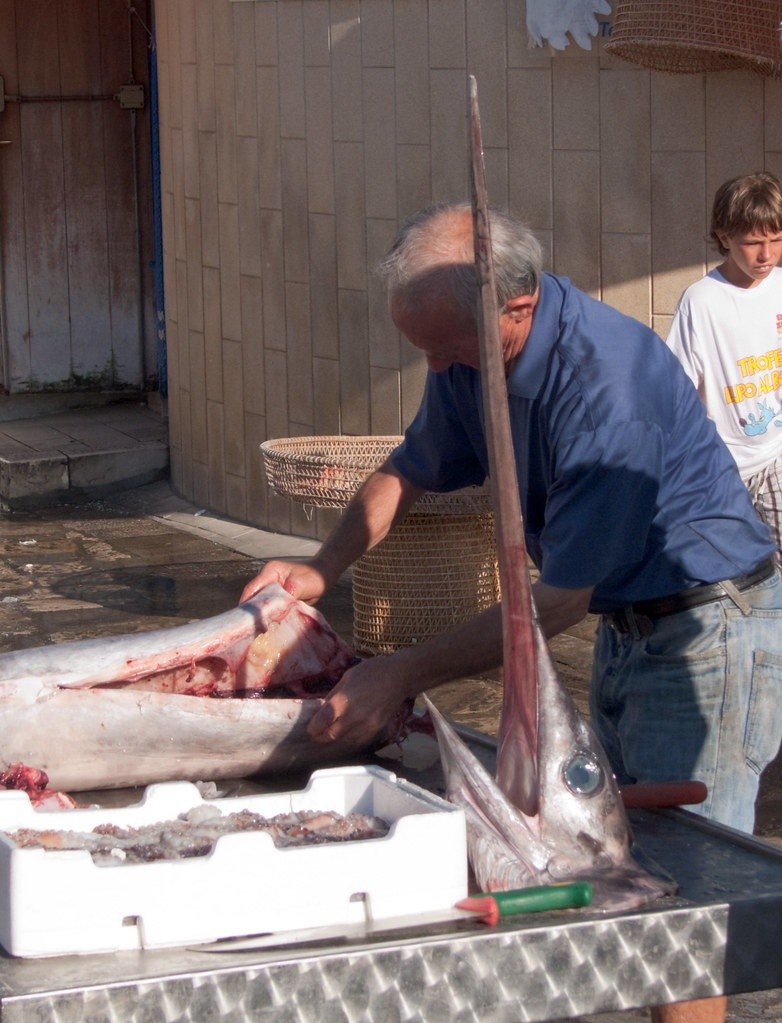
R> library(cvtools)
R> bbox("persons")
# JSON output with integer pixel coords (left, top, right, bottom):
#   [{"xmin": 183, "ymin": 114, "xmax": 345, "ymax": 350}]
[
  {"xmin": 239, "ymin": 201, "xmax": 781, "ymax": 839},
  {"xmin": 662, "ymin": 171, "xmax": 782, "ymax": 559}
]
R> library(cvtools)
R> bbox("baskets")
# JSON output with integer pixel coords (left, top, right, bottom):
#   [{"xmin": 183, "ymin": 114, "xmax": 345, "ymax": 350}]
[{"xmin": 258, "ymin": 435, "xmax": 505, "ymax": 658}]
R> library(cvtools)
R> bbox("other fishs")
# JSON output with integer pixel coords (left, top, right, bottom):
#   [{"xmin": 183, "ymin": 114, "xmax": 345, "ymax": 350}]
[
  {"xmin": 1, "ymin": 584, "xmax": 419, "ymax": 790},
  {"xmin": 4, "ymin": 802, "xmax": 391, "ymax": 869}
]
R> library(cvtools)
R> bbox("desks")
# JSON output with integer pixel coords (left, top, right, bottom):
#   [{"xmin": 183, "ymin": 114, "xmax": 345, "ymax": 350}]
[{"xmin": 0, "ymin": 709, "xmax": 781, "ymax": 1023}]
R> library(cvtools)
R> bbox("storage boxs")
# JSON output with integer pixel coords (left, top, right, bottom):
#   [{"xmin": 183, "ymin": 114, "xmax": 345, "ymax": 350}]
[{"xmin": 0, "ymin": 764, "xmax": 470, "ymax": 958}]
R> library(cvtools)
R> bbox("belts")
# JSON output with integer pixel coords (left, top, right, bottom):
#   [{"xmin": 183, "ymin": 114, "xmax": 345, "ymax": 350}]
[{"xmin": 601, "ymin": 554, "xmax": 775, "ymax": 636}]
